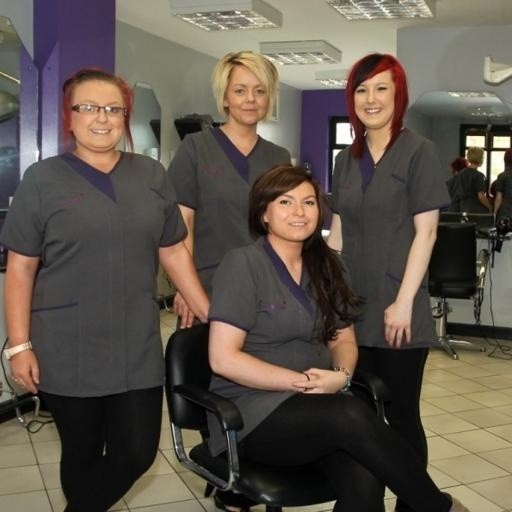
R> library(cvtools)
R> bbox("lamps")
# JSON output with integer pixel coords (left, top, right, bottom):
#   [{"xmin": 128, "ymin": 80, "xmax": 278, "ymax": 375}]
[
  {"xmin": 313, "ymin": 67, "xmax": 360, "ymax": 88},
  {"xmin": 327, "ymin": 0, "xmax": 441, "ymax": 23},
  {"xmin": 170, "ymin": 1, "xmax": 283, "ymax": 33},
  {"xmin": 258, "ymin": 40, "xmax": 341, "ymax": 66}
]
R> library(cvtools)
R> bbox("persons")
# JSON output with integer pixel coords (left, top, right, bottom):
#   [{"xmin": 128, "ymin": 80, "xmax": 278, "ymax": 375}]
[
  {"xmin": 490, "ymin": 148, "xmax": 512, "ymax": 230},
  {"xmin": 0, "ymin": 66, "xmax": 211, "ymax": 512},
  {"xmin": 449, "ymin": 156, "xmax": 469, "ymax": 175},
  {"xmin": 162, "ymin": 49, "xmax": 292, "ymax": 332},
  {"xmin": 324, "ymin": 52, "xmax": 452, "ymax": 512},
  {"xmin": 446, "ymin": 146, "xmax": 493, "ymax": 212},
  {"xmin": 206, "ymin": 160, "xmax": 472, "ymax": 512}
]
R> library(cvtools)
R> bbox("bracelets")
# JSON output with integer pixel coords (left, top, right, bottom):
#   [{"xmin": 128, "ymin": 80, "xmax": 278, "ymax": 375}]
[
  {"xmin": 303, "ymin": 372, "xmax": 310, "ymax": 392},
  {"xmin": 332, "ymin": 365, "xmax": 352, "ymax": 394},
  {"xmin": 2, "ymin": 341, "xmax": 35, "ymax": 363}
]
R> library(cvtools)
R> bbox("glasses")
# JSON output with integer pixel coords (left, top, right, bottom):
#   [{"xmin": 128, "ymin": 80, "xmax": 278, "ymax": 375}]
[{"xmin": 70, "ymin": 103, "xmax": 127, "ymax": 114}]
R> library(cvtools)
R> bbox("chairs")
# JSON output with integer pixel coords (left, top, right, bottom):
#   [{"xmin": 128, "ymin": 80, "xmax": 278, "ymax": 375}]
[
  {"xmin": 162, "ymin": 320, "xmax": 393, "ymax": 511},
  {"xmin": 424, "ymin": 217, "xmax": 488, "ymax": 360}
]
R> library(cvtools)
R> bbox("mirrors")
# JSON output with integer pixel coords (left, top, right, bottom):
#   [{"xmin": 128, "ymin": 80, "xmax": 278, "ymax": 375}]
[
  {"xmin": 123, "ymin": 83, "xmax": 160, "ymax": 164},
  {"xmin": 401, "ymin": 91, "xmax": 512, "ymax": 240},
  {"xmin": 1, "ymin": 16, "xmax": 42, "ymax": 274}
]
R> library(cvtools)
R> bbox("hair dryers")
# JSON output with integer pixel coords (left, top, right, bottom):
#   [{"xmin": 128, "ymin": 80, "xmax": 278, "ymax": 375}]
[{"xmin": 492, "ymin": 216, "xmax": 511, "ymax": 253}]
